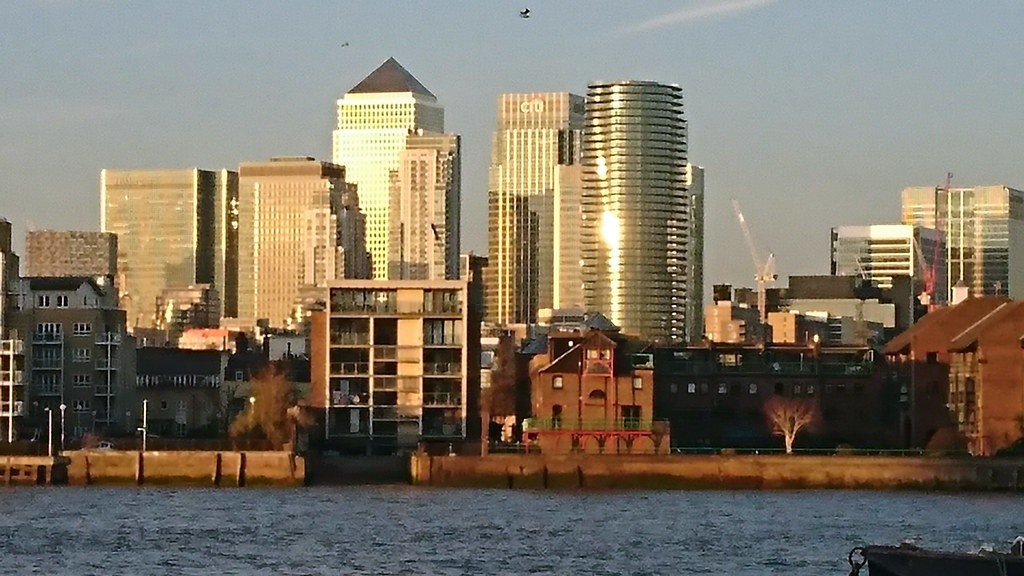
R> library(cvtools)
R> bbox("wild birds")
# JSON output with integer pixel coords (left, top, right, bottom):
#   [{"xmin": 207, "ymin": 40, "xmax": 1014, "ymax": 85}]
[
  {"xmin": 519, "ymin": 8, "xmax": 531, "ymax": 18},
  {"xmin": 337, "ymin": 40, "xmax": 349, "ymax": 49}
]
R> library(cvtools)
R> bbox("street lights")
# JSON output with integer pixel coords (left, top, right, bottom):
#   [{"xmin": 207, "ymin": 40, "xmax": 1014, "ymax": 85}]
[
  {"xmin": 60, "ymin": 405, "xmax": 65, "ymax": 450},
  {"xmin": 44, "ymin": 408, "xmax": 52, "ymax": 457},
  {"xmin": 248, "ymin": 397, "xmax": 255, "ymax": 413},
  {"xmin": 143, "ymin": 400, "xmax": 149, "ymax": 453}
]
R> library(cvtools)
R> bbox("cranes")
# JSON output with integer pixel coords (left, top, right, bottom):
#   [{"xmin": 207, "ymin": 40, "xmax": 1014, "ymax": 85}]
[
  {"xmin": 729, "ymin": 198, "xmax": 777, "ymax": 325},
  {"xmin": 919, "ymin": 172, "xmax": 954, "ymax": 313}
]
